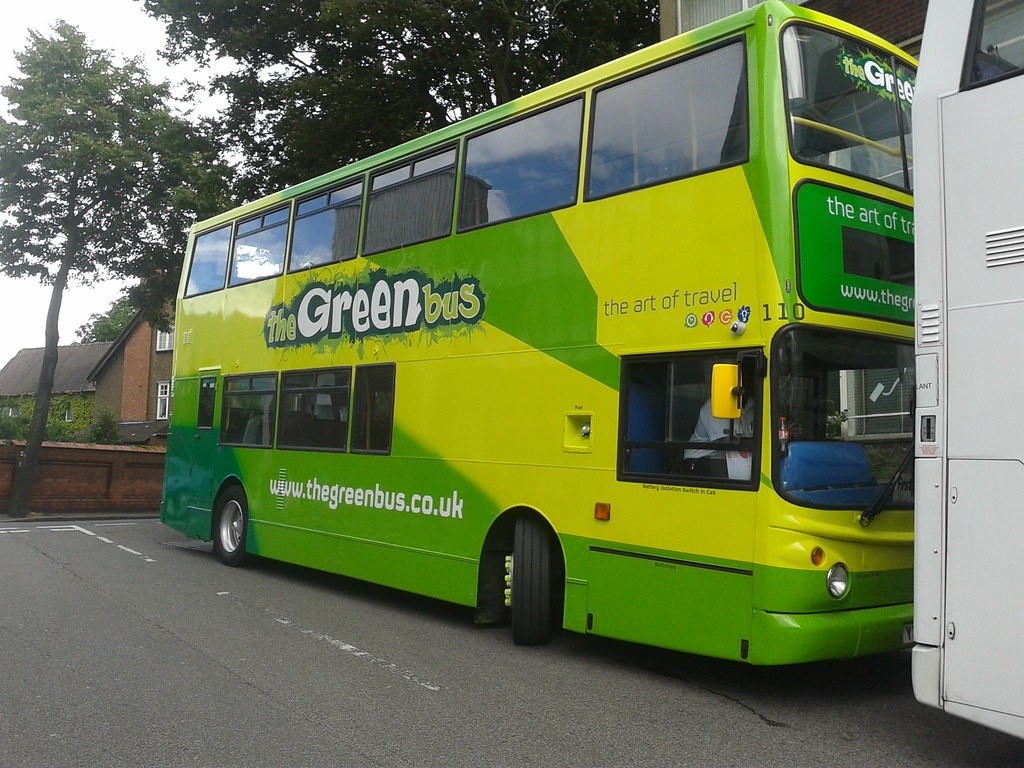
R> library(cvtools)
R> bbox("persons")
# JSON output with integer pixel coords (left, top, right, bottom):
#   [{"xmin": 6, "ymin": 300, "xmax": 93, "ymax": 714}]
[{"xmin": 682, "ymin": 354, "xmax": 807, "ymax": 481}]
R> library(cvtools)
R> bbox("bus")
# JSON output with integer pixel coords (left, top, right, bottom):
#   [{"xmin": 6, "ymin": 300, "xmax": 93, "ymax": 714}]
[
  {"xmin": 908, "ymin": 0, "xmax": 1023, "ymax": 744},
  {"xmin": 158, "ymin": 1, "xmax": 918, "ymax": 669}
]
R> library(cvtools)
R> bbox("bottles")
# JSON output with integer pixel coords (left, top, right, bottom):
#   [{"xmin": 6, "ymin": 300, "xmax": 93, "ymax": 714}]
[{"xmin": 778, "ymin": 416, "xmax": 789, "ymax": 453}]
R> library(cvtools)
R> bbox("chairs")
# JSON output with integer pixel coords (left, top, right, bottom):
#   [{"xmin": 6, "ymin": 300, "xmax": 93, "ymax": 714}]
[{"xmin": 227, "ymin": 392, "xmax": 347, "ymax": 446}]
